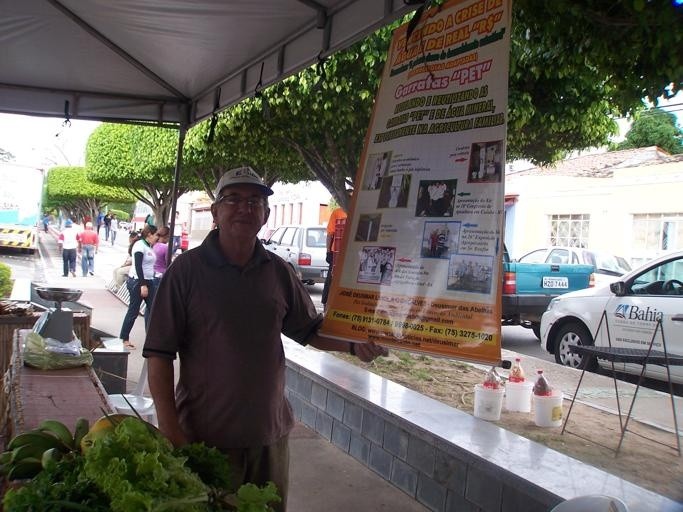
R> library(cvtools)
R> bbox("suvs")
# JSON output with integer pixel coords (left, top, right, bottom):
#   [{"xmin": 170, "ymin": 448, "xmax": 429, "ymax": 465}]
[{"xmin": 260, "ymin": 225, "xmax": 330, "ymax": 286}]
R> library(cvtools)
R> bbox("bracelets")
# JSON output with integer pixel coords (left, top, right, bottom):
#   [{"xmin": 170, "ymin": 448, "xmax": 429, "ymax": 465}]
[{"xmin": 350, "ymin": 342, "xmax": 356, "ymax": 355}]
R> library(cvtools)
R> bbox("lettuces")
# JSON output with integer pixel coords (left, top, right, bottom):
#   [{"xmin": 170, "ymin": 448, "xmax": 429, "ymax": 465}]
[{"xmin": 1, "ymin": 416, "xmax": 283, "ymax": 511}]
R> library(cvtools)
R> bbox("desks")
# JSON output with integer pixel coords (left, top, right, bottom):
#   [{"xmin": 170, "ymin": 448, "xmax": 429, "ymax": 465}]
[
  {"xmin": 559, "ymin": 310, "xmax": 681, "ymax": 459},
  {"xmin": 6, "ymin": 325, "xmax": 125, "ymax": 484}
]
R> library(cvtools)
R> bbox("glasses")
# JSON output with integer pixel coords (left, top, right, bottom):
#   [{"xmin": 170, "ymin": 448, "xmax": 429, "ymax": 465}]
[{"xmin": 216, "ymin": 193, "xmax": 262, "ymax": 206}]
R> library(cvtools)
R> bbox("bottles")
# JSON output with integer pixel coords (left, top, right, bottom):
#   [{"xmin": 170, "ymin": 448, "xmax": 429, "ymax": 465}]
[
  {"xmin": 483, "ymin": 367, "xmax": 501, "ymax": 389},
  {"xmin": 533, "ymin": 368, "xmax": 551, "ymax": 396},
  {"xmin": 508, "ymin": 357, "xmax": 525, "ymax": 382}
]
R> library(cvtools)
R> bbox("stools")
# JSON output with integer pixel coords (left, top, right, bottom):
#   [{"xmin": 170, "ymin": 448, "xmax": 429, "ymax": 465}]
[{"xmin": 103, "ymin": 391, "xmax": 157, "ymax": 430}]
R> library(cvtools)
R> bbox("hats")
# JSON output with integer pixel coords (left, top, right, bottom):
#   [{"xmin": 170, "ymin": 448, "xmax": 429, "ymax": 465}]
[{"xmin": 216, "ymin": 166, "xmax": 274, "ymax": 197}]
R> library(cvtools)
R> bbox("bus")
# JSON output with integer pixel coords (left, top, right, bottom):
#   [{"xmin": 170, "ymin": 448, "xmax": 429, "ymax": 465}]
[{"xmin": 0, "ymin": 158, "xmax": 44, "ymax": 254}]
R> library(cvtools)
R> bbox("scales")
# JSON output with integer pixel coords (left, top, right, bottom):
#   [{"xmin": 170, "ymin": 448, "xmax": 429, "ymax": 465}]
[{"xmin": 32, "ymin": 287, "xmax": 83, "ymax": 343}]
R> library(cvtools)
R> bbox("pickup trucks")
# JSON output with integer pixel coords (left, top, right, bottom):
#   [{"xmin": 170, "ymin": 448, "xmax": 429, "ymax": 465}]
[{"xmin": 501, "ymin": 243, "xmax": 596, "ymax": 340}]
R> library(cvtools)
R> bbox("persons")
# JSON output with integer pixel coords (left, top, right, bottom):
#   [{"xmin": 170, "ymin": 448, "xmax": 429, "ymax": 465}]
[
  {"xmin": 59, "ymin": 209, "xmax": 118, "ymax": 277},
  {"xmin": 113, "ymin": 232, "xmax": 139, "ymax": 293},
  {"xmin": 119, "ymin": 224, "xmax": 154, "ymax": 349},
  {"xmin": 151, "ymin": 227, "xmax": 171, "ymax": 296},
  {"xmin": 42, "ymin": 214, "xmax": 49, "ymax": 233},
  {"xmin": 359, "ymin": 146, "xmax": 499, "ymax": 282},
  {"xmin": 172, "ymin": 211, "xmax": 186, "ymax": 257},
  {"xmin": 321, "ymin": 189, "xmax": 353, "ymax": 312},
  {"xmin": 143, "ymin": 167, "xmax": 389, "ymax": 512}
]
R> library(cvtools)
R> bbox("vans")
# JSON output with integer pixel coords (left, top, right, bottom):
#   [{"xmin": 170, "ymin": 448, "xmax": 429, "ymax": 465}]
[{"xmin": 130, "ymin": 199, "xmax": 189, "ymax": 253}]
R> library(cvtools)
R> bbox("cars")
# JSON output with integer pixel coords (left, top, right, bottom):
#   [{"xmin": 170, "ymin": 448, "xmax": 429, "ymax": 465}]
[
  {"xmin": 515, "ymin": 247, "xmax": 649, "ymax": 287},
  {"xmin": 118, "ymin": 222, "xmax": 129, "ymax": 228},
  {"xmin": 539, "ymin": 251, "xmax": 683, "ymax": 391}
]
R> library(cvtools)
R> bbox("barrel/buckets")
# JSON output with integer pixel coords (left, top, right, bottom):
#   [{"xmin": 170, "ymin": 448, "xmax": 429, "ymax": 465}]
[
  {"xmin": 473, "ymin": 384, "xmax": 505, "ymax": 421},
  {"xmin": 533, "ymin": 389, "xmax": 564, "ymax": 427},
  {"xmin": 504, "ymin": 380, "xmax": 534, "ymax": 413}
]
